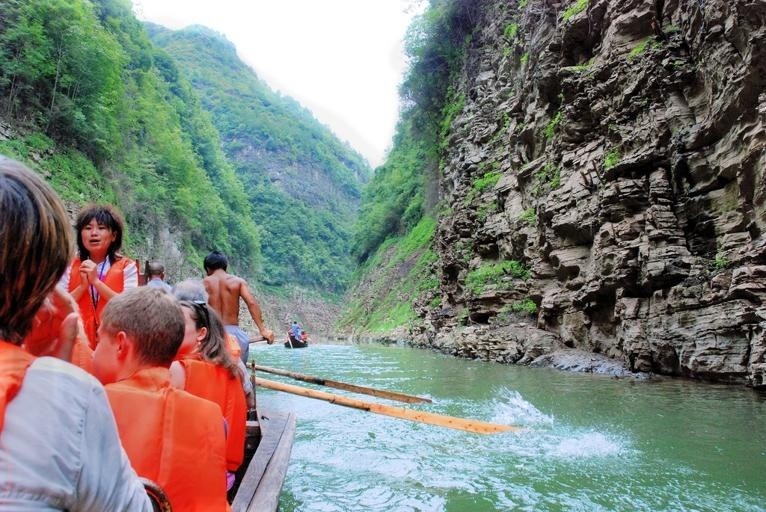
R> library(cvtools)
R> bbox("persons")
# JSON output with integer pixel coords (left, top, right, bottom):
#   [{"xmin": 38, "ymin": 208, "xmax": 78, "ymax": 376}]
[
  {"xmin": 0, "ymin": 155, "xmax": 171, "ymax": 512},
  {"xmin": 91, "ymin": 286, "xmax": 227, "ymax": 512},
  {"xmin": 169, "ymin": 303, "xmax": 256, "ymax": 473},
  {"xmin": 286, "ymin": 320, "xmax": 309, "ymax": 342},
  {"xmin": 172, "ymin": 281, "xmax": 210, "ymax": 305},
  {"xmin": 64, "ymin": 205, "xmax": 138, "ymax": 352},
  {"xmin": 203, "ymin": 251, "xmax": 274, "ymax": 363},
  {"xmin": 147, "ymin": 262, "xmax": 169, "ymax": 286}
]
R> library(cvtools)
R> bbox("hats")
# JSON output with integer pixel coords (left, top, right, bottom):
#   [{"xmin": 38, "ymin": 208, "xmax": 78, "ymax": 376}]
[{"xmin": 172, "ymin": 280, "xmax": 207, "ymax": 306}]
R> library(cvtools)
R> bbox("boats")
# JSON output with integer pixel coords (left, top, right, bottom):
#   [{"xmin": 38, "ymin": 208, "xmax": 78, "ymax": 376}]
[
  {"xmin": 206, "ymin": 360, "xmax": 297, "ymax": 512},
  {"xmin": 228, "ymin": 410, "xmax": 295, "ymax": 510}
]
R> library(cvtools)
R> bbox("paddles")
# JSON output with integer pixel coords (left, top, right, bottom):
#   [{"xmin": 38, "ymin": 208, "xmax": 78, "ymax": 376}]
[
  {"xmin": 247, "ymin": 362, "xmax": 433, "ymax": 405},
  {"xmin": 247, "ymin": 376, "xmax": 514, "ymax": 435}
]
